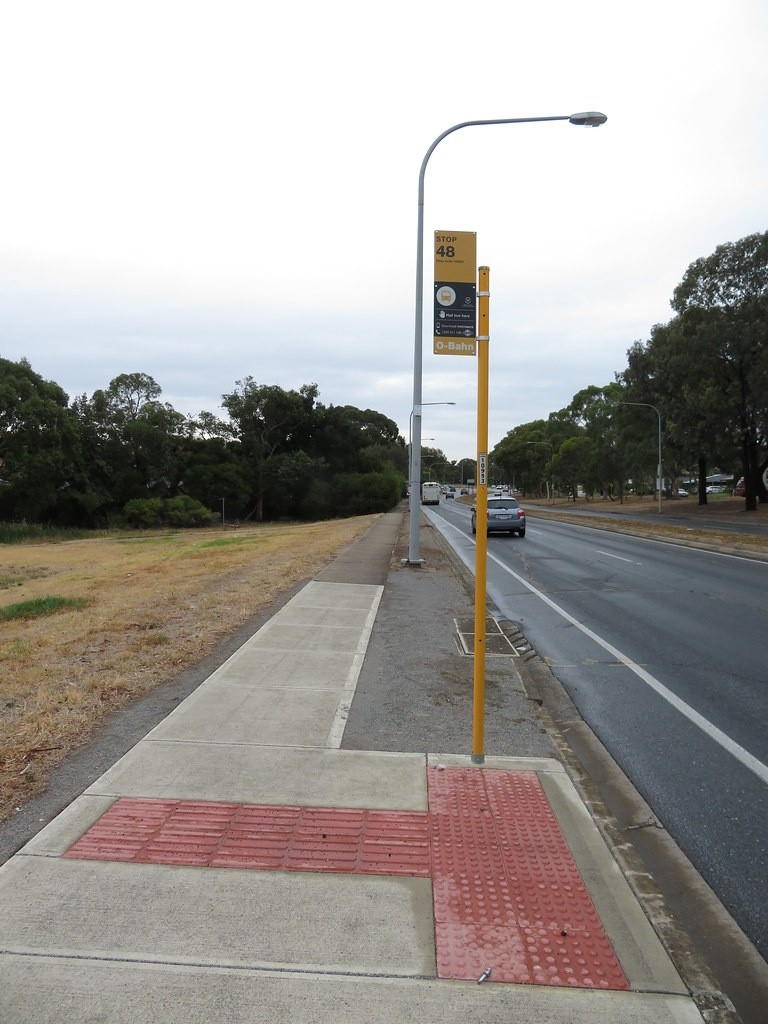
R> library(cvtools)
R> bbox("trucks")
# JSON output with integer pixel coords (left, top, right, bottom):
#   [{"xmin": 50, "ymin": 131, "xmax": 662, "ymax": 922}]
[{"xmin": 735, "ymin": 476, "xmax": 746, "ymax": 497}]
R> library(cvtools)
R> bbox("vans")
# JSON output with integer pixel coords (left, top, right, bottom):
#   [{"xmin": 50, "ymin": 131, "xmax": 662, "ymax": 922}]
[{"xmin": 443, "ymin": 485, "xmax": 453, "ymax": 490}]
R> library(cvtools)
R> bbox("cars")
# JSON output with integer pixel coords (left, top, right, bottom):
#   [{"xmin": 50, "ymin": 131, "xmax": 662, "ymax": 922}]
[
  {"xmin": 470, "ymin": 496, "xmax": 526, "ymax": 538},
  {"xmin": 445, "ymin": 492, "xmax": 454, "ymax": 499},
  {"xmin": 496, "ymin": 485, "xmax": 504, "ymax": 489},
  {"xmin": 491, "ymin": 485, "xmax": 496, "ymax": 489},
  {"xmin": 513, "ymin": 489, "xmax": 518, "ymax": 492},
  {"xmin": 494, "ymin": 491, "xmax": 502, "ymax": 496},
  {"xmin": 443, "ymin": 488, "xmax": 448, "ymax": 494},
  {"xmin": 450, "ymin": 487, "xmax": 456, "ymax": 492},
  {"xmin": 672, "ymin": 489, "xmax": 688, "ymax": 497},
  {"xmin": 706, "ymin": 486, "xmax": 726, "ymax": 494},
  {"xmin": 503, "ymin": 488, "xmax": 508, "ymax": 492},
  {"xmin": 461, "ymin": 489, "xmax": 468, "ymax": 495}
]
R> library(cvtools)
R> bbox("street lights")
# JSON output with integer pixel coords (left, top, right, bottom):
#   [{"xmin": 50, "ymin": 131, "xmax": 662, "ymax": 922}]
[
  {"xmin": 408, "ymin": 111, "xmax": 607, "ymax": 567},
  {"xmin": 429, "ymin": 463, "xmax": 449, "ymax": 482},
  {"xmin": 526, "ymin": 442, "xmax": 555, "ymax": 505},
  {"xmin": 409, "ymin": 402, "xmax": 457, "ymax": 510},
  {"xmin": 613, "ymin": 402, "xmax": 662, "ymax": 514}
]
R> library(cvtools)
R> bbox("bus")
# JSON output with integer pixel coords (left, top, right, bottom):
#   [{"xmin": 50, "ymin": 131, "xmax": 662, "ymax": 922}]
[{"xmin": 422, "ymin": 482, "xmax": 440, "ymax": 505}]
